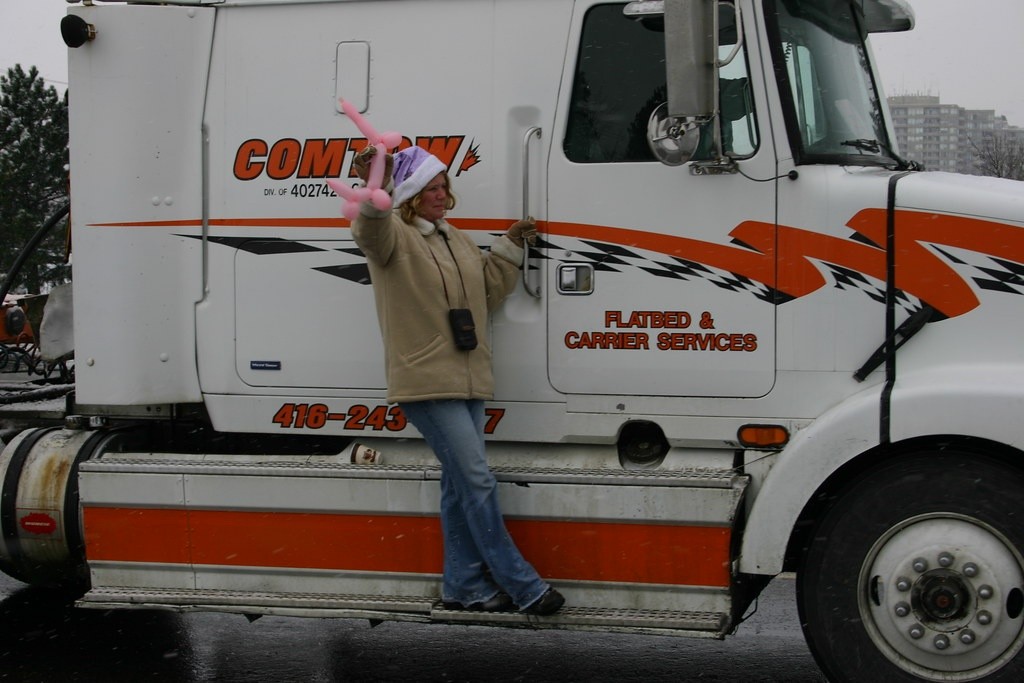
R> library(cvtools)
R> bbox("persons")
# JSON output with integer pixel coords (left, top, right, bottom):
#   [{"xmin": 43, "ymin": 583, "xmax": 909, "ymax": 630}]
[{"xmin": 353, "ymin": 145, "xmax": 568, "ymax": 616}]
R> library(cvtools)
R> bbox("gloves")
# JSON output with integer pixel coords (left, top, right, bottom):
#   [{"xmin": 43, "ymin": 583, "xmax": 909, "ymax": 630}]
[
  {"xmin": 352, "ymin": 144, "xmax": 394, "ymax": 189},
  {"xmin": 507, "ymin": 215, "xmax": 537, "ymax": 249}
]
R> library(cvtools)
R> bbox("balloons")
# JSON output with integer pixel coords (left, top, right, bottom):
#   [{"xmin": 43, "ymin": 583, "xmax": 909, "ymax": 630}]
[{"xmin": 328, "ymin": 100, "xmax": 401, "ymax": 219}]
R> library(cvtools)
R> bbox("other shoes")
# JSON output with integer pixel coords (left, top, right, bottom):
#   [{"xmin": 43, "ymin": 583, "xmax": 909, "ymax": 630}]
[
  {"xmin": 443, "ymin": 590, "xmax": 514, "ymax": 612},
  {"xmin": 529, "ymin": 586, "xmax": 566, "ymax": 616}
]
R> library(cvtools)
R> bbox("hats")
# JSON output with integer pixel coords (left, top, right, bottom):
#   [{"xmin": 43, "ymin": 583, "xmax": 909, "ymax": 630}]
[{"xmin": 390, "ymin": 146, "xmax": 447, "ymax": 209}]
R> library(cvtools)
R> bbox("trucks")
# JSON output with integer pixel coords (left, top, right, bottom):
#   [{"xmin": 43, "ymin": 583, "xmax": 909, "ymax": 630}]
[{"xmin": 0, "ymin": 0, "xmax": 1024, "ymax": 682}]
